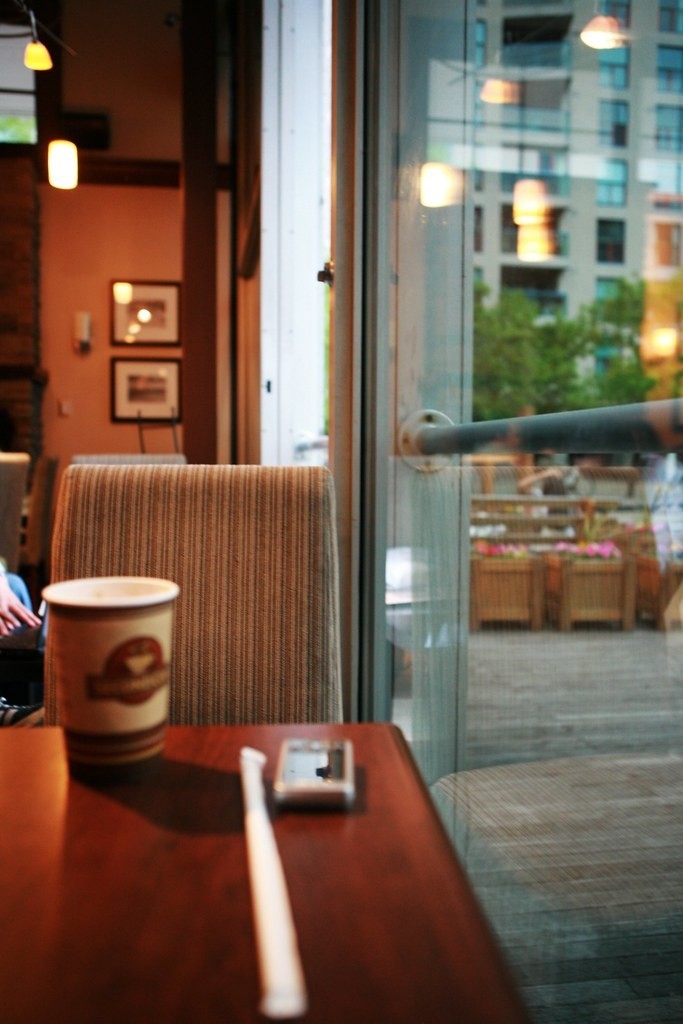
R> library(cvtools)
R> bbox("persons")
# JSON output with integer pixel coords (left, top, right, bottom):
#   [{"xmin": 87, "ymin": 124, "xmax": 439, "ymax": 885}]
[
  {"xmin": 516, "ymin": 465, "xmax": 581, "ymax": 541},
  {"xmin": 0, "ymin": 556, "xmax": 49, "ymax": 728}
]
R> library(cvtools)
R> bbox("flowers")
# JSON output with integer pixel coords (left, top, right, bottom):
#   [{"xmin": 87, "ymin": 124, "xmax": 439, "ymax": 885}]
[{"xmin": 473, "ymin": 539, "xmax": 619, "ymax": 561}]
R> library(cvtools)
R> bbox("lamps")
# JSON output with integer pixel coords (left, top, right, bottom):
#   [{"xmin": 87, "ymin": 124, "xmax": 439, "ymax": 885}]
[
  {"xmin": 415, "ymin": 11, "xmax": 682, "ymax": 378},
  {"xmin": 23, "ymin": 10, "xmax": 79, "ymax": 72},
  {"xmin": 46, "ymin": 138, "xmax": 78, "ymax": 190}
]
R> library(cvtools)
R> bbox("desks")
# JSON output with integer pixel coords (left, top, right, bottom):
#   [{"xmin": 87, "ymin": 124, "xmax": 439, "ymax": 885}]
[{"xmin": 0, "ymin": 722, "xmax": 532, "ymax": 1024}]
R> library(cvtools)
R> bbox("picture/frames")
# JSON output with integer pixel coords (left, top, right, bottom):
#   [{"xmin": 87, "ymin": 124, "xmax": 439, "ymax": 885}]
[
  {"xmin": 109, "ymin": 356, "xmax": 182, "ymax": 425},
  {"xmin": 109, "ymin": 277, "xmax": 180, "ymax": 345}
]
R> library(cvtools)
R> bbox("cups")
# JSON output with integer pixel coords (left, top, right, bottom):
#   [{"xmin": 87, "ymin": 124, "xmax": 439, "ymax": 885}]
[{"xmin": 42, "ymin": 576, "xmax": 183, "ymax": 768}]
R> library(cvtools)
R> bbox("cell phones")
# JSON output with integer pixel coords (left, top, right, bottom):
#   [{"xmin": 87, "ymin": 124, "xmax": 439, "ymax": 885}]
[{"xmin": 272, "ymin": 739, "xmax": 356, "ymax": 810}]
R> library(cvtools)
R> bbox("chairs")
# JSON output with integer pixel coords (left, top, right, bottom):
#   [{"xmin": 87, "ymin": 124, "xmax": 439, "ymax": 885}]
[{"xmin": 42, "ymin": 463, "xmax": 343, "ymax": 722}]
